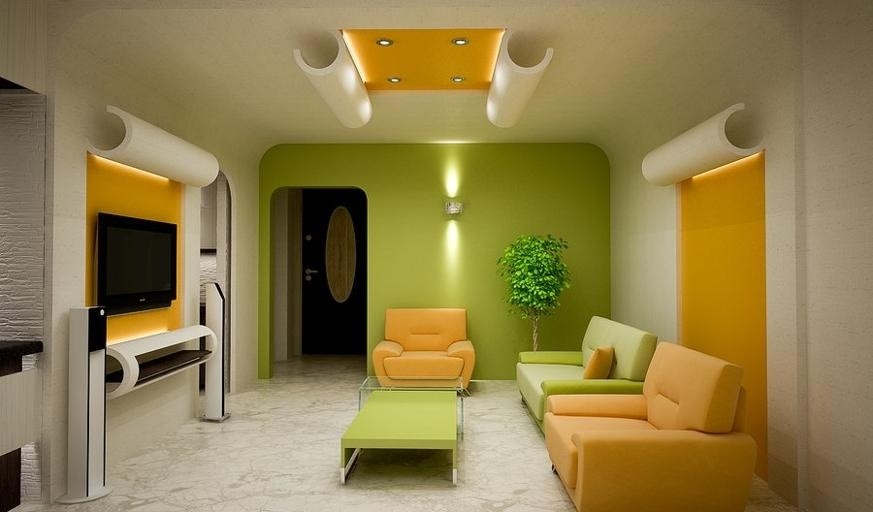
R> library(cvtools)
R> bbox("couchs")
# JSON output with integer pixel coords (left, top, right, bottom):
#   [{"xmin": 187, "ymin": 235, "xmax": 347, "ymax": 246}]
[
  {"xmin": 373, "ymin": 308, "xmax": 475, "ymax": 397},
  {"xmin": 516, "ymin": 316, "xmax": 658, "ymax": 436},
  {"xmin": 545, "ymin": 341, "xmax": 758, "ymax": 512}
]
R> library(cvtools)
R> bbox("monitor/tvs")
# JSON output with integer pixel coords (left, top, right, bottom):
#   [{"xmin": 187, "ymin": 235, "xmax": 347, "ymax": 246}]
[{"xmin": 97, "ymin": 212, "xmax": 177, "ymax": 318}]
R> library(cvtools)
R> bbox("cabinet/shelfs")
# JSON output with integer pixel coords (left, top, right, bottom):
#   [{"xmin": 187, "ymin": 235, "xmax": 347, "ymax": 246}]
[{"xmin": 0, "ymin": 341, "xmax": 43, "ymax": 512}]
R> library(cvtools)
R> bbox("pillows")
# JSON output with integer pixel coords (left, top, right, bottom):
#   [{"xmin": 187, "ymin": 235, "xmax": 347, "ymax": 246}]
[{"xmin": 583, "ymin": 344, "xmax": 614, "ymax": 379}]
